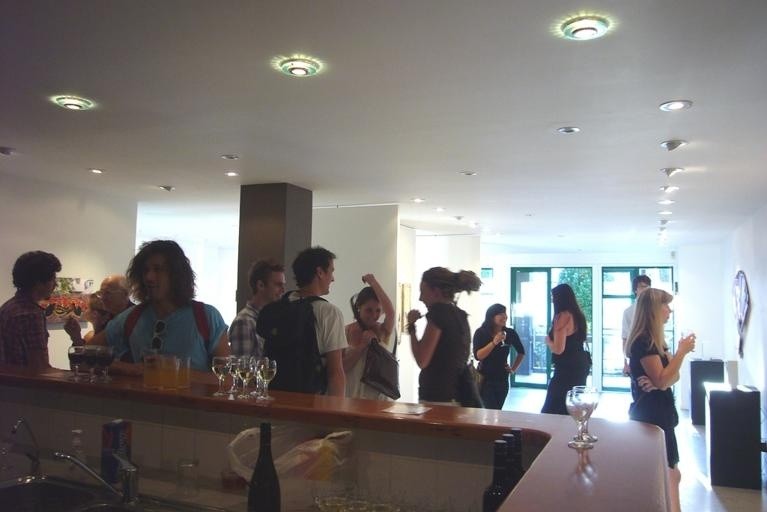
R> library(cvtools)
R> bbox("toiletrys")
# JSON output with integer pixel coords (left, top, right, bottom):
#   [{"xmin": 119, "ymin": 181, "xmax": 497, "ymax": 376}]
[{"xmin": 65, "ymin": 427, "xmax": 89, "ymax": 474}]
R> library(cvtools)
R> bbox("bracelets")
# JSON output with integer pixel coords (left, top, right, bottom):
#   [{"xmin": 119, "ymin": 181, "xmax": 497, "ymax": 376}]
[
  {"xmin": 491, "ymin": 339, "xmax": 497, "ymax": 347},
  {"xmin": 406, "ymin": 321, "xmax": 415, "ymax": 334}
]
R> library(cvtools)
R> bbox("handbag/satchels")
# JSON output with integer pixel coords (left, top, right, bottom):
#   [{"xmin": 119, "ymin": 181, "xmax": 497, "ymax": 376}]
[
  {"xmin": 584, "ymin": 347, "xmax": 594, "ymax": 371},
  {"xmin": 358, "ymin": 342, "xmax": 403, "ymax": 401},
  {"xmin": 464, "ymin": 361, "xmax": 486, "ymax": 409}
]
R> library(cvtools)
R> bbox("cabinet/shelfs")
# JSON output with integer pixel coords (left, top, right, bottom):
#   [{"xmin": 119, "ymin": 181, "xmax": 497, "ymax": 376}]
[
  {"xmin": 692, "ymin": 359, "xmax": 724, "ymax": 427},
  {"xmin": 704, "ymin": 382, "xmax": 761, "ymax": 491}
]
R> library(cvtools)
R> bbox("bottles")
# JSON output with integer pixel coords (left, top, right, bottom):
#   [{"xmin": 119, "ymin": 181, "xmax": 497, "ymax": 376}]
[
  {"xmin": 483, "ymin": 427, "xmax": 526, "ymax": 512},
  {"xmin": 248, "ymin": 422, "xmax": 281, "ymax": 512}
]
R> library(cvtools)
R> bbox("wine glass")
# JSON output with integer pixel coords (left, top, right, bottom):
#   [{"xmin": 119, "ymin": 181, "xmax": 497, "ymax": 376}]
[
  {"xmin": 498, "ymin": 331, "xmax": 509, "ymax": 347},
  {"xmin": 67, "ymin": 344, "xmax": 113, "ymax": 385},
  {"xmin": 565, "ymin": 385, "xmax": 600, "ymax": 450},
  {"xmin": 212, "ymin": 355, "xmax": 277, "ymax": 402}
]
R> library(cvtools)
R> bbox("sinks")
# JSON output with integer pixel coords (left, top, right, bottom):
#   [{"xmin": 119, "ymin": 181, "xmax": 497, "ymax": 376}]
[
  {"xmin": 83, "ymin": 501, "xmax": 184, "ymax": 512},
  {"xmin": 1, "ymin": 475, "xmax": 106, "ymax": 512}
]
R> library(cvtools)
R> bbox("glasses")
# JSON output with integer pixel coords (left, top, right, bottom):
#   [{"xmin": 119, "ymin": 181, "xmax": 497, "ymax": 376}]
[
  {"xmin": 148, "ymin": 317, "xmax": 167, "ymax": 351},
  {"xmin": 92, "ymin": 289, "xmax": 119, "ymax": 297}
]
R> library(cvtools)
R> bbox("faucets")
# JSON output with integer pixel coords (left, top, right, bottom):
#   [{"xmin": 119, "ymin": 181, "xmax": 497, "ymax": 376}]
[
  {"xmin": 51, "ymin": 449, "xmax": 139, "ymax": 502},
  {"xmin": 13, "ymin": 415, "xmax": 40, "ymax": 474}
]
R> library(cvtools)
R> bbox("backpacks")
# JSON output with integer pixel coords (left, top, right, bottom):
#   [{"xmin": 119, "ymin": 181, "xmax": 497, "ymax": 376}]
[{"xmin": 256, "ymin": 290, "xmax": 329, "ymax": 392}]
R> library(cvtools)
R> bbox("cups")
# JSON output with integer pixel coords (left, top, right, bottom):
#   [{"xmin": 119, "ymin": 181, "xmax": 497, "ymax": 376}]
[{"xmin": 143, "ymin": 355, "xmax": 192, "ymax": 391}]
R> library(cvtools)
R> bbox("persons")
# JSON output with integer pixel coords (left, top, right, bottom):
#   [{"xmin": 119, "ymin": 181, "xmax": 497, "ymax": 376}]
[
  {"xmin": 540, "ymin": 283, "xmax": 592, "ymax": 417},
  {"xmin": 406, "ymin": 266, "xmax": 486, "ymax": 407},
  {"xmin": 0, "ymin": 250, "xmax": 63, "ymax": 370},
  {"xmin": 82, "ymin": 291, "xmax": 112, "ymax": 343},
  {"xmin": 621, "ymin": 274, "xmax": 651, "ymax": 377},
  {"xmin": 340, "ymin": 272, "xmax": 395, "ymax": 401},
  {"xmin": 226, "ymin": 260, "xmax": 287, "ymax": 380},
  {"xmin": 62, "ymin": 274, "xmax": 136, "ymax": 368},
  {"xmin": 472, "ymin": 303, "xmax": 525, "ymax": 411},
  {"xmin": 623, "ymin": 287, "xmax": 695, "ymax": 511},
  {"xmin": 76, "ymin": 239, "xmax": 230, "ymax": 377},
  {"xmin": 256, "ymin": 245, "xmax": 349, "ymax": 397}
]
